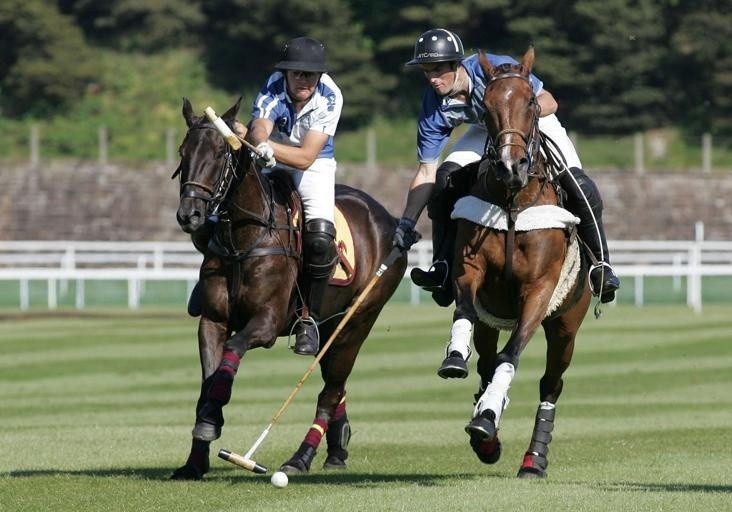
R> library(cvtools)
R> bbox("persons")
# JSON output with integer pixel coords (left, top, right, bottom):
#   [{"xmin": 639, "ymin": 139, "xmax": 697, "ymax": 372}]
[
  {"xmin": 233, "ymin": 36, "xmax": 345, "ymax": 355},
  {"xmin": 391, "ymin": 27, "xmax": 621, "ymax": 308}
]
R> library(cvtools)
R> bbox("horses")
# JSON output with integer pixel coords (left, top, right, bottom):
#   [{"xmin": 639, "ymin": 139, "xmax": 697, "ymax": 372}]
[
  {"xmin": 168, "ymin": 95, "xmax": 423, "ymax": 481},
  {"xmin": 436, "ymin": 46, "xmax": 595, "ymax": 481}
]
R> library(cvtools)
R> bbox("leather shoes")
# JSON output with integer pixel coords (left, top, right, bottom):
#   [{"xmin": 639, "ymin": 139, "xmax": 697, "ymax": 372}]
[
  {"xmin": 589, "ymin": 263, "xmax": 620, "ymax": 303},
  {"xmin": 411, "ymin": 267, "xmax": 455, "ymax": 307},
  {"xmin": 294, "ymin": 324, "xmax": 319, "ymax": 355}
]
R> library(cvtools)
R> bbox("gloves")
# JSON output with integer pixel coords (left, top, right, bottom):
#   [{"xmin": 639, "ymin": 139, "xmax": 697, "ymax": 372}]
[
  {"xmin": 392, "ymin": 217, "xmax": 422, "ymax": 250},
  {"xmin": 251, "ymin": 142, "xmax": 276, "ymax": 168}
]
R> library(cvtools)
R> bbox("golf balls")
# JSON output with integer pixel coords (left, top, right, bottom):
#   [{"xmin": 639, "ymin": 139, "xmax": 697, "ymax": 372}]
[{"xmin": 271, "ymin": 472, "xmax": 288, "ymax": 488}]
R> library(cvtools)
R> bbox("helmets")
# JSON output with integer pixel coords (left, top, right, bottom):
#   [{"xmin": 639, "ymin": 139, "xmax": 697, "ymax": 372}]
[
  {"xmin": 406, "ymin": 28, "xmax": 465, "ymax": 66},
  {"xmin": 274, "ymin": 37, "xmax": 335, "ymax": 73}
]
